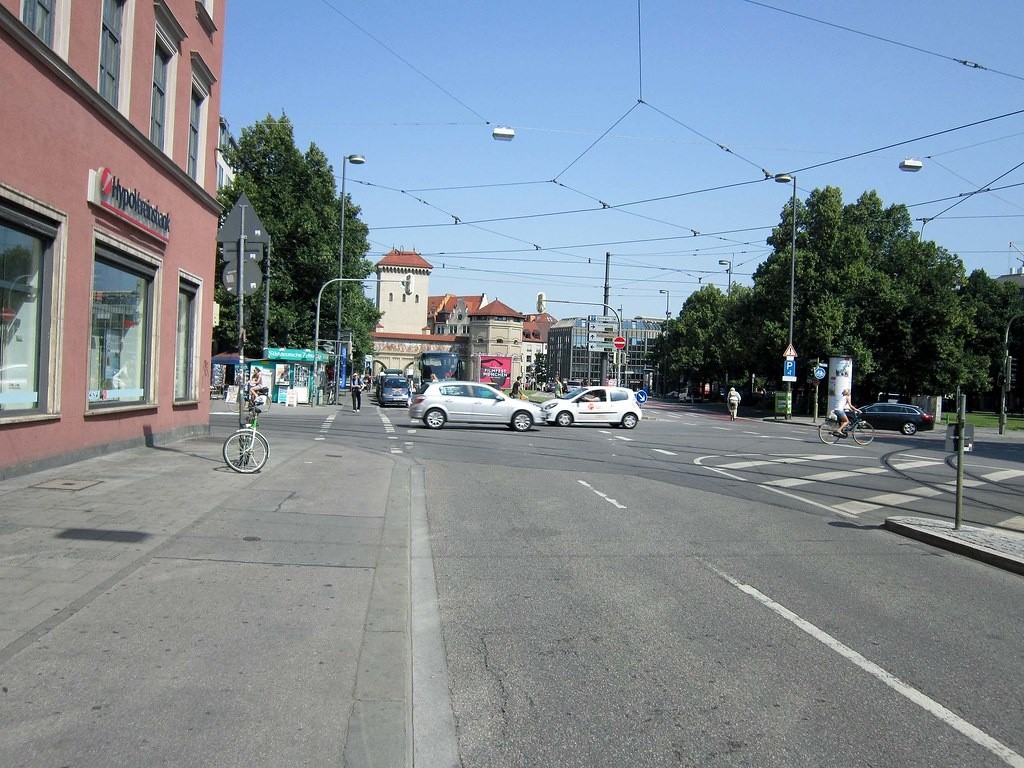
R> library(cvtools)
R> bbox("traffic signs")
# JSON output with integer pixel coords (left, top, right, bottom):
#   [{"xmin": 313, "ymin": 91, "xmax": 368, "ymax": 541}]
[{"xmin": 810, "ymin": 359, "xmax": 828, "ymax": 368}]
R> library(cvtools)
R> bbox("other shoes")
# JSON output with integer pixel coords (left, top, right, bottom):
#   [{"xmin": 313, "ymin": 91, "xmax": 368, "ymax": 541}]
[
  {"xmin": 837, "ymin": 429, "xmax": 844, "ymax": 435},
  {"xmin": 254, "ymin": 396, "xmax": 259, "ymax": 402},
  {"xmin": 353, "ymin": 410, "xmax": 357, "ymax": 413},
  {"xmin": 357, "ymin": 410, "xmax": 361, "ymax": 412}
]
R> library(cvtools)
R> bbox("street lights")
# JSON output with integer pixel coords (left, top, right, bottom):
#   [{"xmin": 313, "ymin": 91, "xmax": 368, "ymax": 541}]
[
  {"xmin": 333, "ymin": 153, "xmax": 365, "ymax": 403},
  {"xmin": 659, "ymin": 290, "xmax": 669, "ymax": 398},
  {"xmin": 718, "ymin": 259, "xmax": 732, "ymax": 404},
  {"xmin": 775, "ymin": 174, "xmax": 795, "ymax": 419}
]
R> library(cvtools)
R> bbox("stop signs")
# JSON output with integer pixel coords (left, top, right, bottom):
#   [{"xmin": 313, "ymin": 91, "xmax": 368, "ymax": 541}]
[{"xmin": 614, "ymin": 337, "xmax": 626, "ymax": 349}]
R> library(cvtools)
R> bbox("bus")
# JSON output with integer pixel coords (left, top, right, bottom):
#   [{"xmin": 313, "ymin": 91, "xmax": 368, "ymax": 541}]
[{"xmin": 419, "ymin": 351, "xmax": 466, "ymax": 390}]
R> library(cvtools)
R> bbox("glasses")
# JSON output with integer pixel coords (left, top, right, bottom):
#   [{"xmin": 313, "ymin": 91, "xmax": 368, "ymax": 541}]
[{"xmin": 253, "ymin": 370, "xmax": 255, "ymax": 371}]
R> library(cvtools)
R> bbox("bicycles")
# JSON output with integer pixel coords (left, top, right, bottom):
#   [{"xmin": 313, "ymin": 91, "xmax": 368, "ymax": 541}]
[
  {"xmin": 227, "ymin": 382, "xmax": 272, "ymax": 413},
  {"xmin": 818, "ymin": 409, "xmax": 875, "ymax": 445},
  {"xmin": 729, "ymin": 403, "xmax": 735, "ymax": 421},
  {"xmin": 223, "ymin": 408, "xmax": 271, "ymax": 474}
]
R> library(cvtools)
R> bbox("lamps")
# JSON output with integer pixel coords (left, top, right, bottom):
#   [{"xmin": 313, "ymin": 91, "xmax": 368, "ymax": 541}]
[
  {"xmin": 899, "ymin": 158, "xmax": 922, "ymax": 172},
  {"xmin": 492, "ymin": 127, "xmax": 515, "ymax": 142}
]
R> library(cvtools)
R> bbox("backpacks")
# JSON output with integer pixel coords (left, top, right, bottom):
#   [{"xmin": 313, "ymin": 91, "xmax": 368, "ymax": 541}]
[{"xmin": 730, "ymin": 391, "xmax": 739, "ymax": 404}]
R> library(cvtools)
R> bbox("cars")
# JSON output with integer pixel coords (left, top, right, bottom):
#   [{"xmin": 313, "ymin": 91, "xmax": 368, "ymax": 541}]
[
  {"xmin": 540, "ymin": 385, "xmax": 642, "ymax": 429},
  {"xmin": 825, "ymin": 402, "xmax": 934, "ymax": 435},
  {"xmin": 374, "ymin": 372, "xmax": 409, "ymax": 407},
  {"xmin": 407, "ymin": 381, "xmax": 547, "ymax": 432},
  {"xmin": 544, "ymin": 383, "xmax": 557, "ymax": 392},
  {"xmin": 666, "ymin": 390, "xmax": 678, "ymax": 399},
  {"xmin": 678, "ymin": 388, "xmax": 702, "ymax": 403}
]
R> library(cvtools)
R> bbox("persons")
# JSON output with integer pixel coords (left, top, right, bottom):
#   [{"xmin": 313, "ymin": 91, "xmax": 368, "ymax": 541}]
[
  {"xmin": 834, "ymin": 388, "xmax": 862, "ymax": 435},
  {"xmin": 582, "ymin": 391, "xmax": 601, "ymax": 402},
  {"xmin": 727, "ymin": 387, "xmax": 741, "ymax": 418},
  {"xmin": 512, "ymin": 376, "xmax": 523, "ymax": 398},
  {"xmin": 549, "ymin": 376, "xmax": 562, "ymax": 398},
  {"xmin": 247, "ymin": 367, "xmax": 263, "ymax": 403},
  {"xmin": 351, "ymin": 373, "xmax": 364, "ymax": 413},
  {"xmin": 404, "ymin": 378, "xmax": 414, "ymax": 409}
]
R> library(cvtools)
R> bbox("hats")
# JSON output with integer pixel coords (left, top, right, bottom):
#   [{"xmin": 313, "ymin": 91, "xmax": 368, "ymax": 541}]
[
  {"xmin": 730, "ymin": 387, "xmax": 735, "ymax": 391},
  {"xmin": 352, "ymin": 373, "xmax": 358, "ymax": 377},
  {"xmin": 843, "ymin": 389, "xmax": 850, "ymax": 396},
  {"xmin": 517, "ymin": 376, "xmax": 523, "ymax": 379}
]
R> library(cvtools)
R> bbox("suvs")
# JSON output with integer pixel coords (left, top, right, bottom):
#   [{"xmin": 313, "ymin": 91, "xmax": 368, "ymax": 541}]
[{"xmin": 563, "ymin": 381, "xmax": 581, "ymax": 393}]
